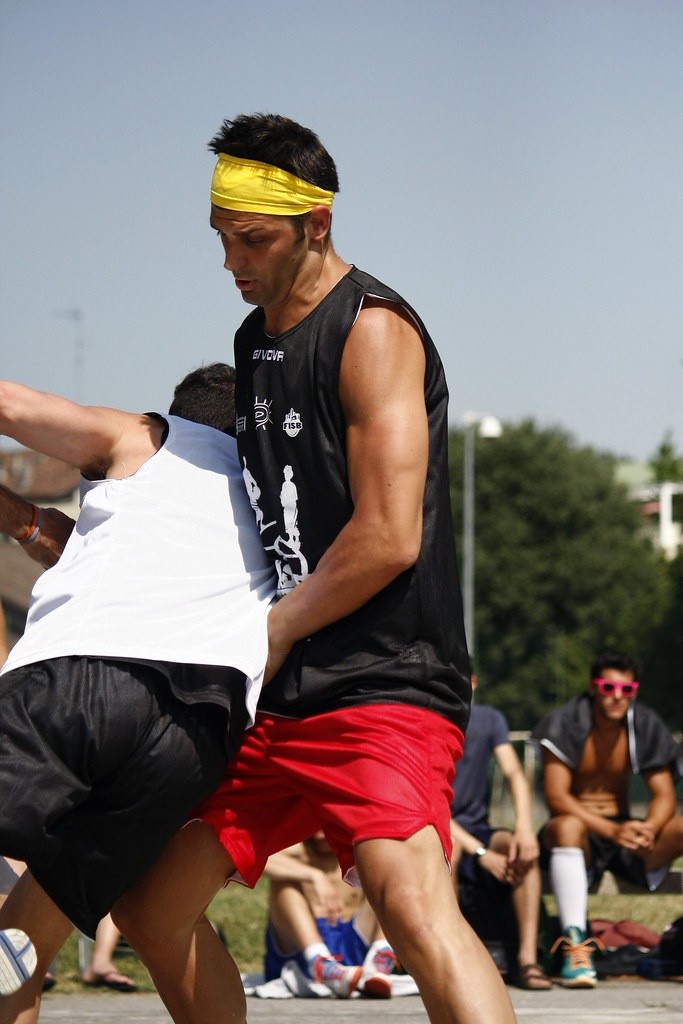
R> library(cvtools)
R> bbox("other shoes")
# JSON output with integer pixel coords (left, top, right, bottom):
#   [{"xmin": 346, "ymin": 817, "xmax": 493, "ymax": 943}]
[{"xmin": 0, "ymin": 928, "xmax": 37, "ymax": 995}]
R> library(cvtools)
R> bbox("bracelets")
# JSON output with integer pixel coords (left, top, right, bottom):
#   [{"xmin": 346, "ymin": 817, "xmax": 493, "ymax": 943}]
[
  {"xmin": 20, "ymin": 506, "xmax": 42, "ymax": 544},
  {"xmin": 15, "ymin": 503, "xmax": 37, "ymax": 542}
]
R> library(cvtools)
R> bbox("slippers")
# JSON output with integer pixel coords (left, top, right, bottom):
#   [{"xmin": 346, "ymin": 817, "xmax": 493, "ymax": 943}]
[
  {"xmin": 83, "ymin": 969, "xmax": 136, "ymax": 992},
  {"xmin": 43, "ymin": 977, "xmax": 54, "ymax": 992}
]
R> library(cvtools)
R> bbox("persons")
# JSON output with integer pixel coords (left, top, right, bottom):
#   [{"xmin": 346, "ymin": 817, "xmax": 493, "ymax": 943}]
[
  {"xmin": 0, "ymin": 363, "xmax": 277, "ymax": 1024},
  {"xmin": 536, "ymin": 648, "xmax": 683, "ymax": 989},
  {"xmin": 261, "ymin": 646, "xmax": 553, "ymax": 998},
  {"xmin": 107, "ymin": 112, "xmax": 517, "ymax": 1024}
]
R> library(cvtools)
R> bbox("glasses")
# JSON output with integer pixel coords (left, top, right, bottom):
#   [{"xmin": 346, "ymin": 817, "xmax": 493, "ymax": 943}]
[{"xmin": 592, "ymin": 679, "xmax": 639, "ymax": 697}]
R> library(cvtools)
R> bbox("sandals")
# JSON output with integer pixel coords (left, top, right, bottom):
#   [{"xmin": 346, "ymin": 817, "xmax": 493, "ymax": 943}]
[{"xmin": 519, "ymin": 964, "xmax": 553, "ymax": 990}]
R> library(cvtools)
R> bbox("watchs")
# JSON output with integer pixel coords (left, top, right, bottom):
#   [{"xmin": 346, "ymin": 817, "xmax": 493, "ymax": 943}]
[{"xmin": 471, "ymin": 845, "xmax": 488, "ymax": 862}]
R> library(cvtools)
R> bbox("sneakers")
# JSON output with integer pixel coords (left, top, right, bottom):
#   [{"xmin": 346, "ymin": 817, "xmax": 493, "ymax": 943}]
[
  {"xmin": 552, "ymin": 926, "xmax": 605, "ymax": 987},
  {"xmin": 311, "ymin": 956, "xmax": 363, "ymax": 997},
  {"xmin": 356, "ymin": 949, "xmax": 399, "ymax": 998}
]
